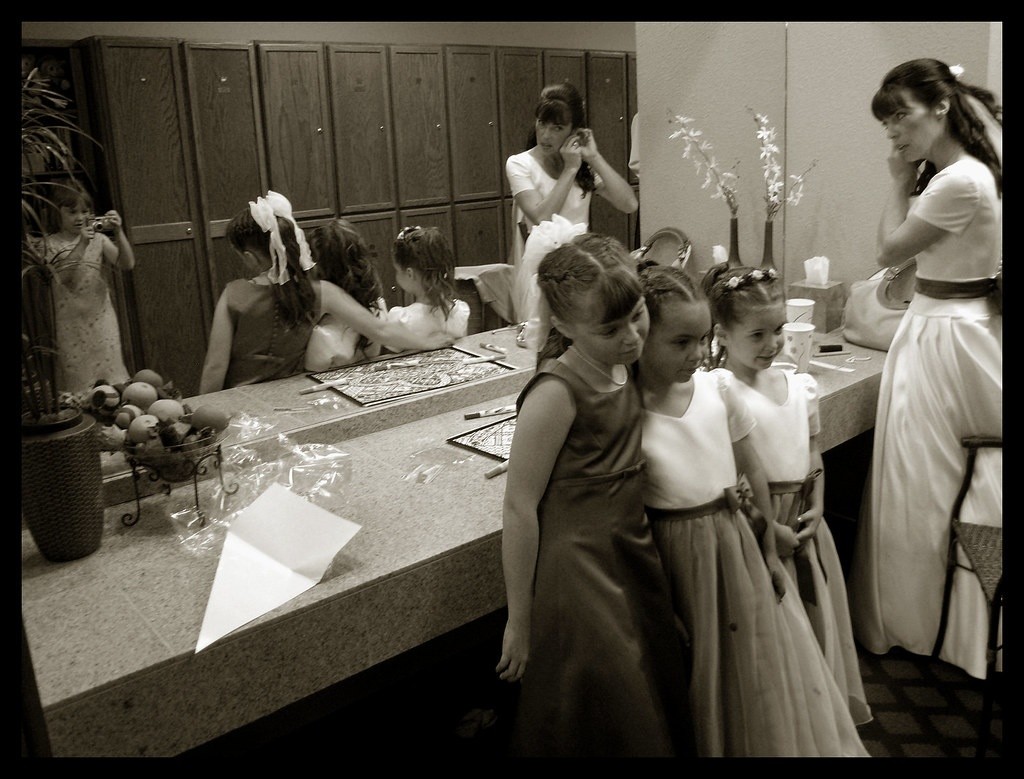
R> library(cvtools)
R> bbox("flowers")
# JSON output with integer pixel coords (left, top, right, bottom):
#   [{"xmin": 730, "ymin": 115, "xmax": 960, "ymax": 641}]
[
  {"xmin": 743, "ymin": 102, "xmax": 817, "ymax": 221},
  {"xmin": 667, "ymin": 113, "xmax": 742, "ymax": 217}
]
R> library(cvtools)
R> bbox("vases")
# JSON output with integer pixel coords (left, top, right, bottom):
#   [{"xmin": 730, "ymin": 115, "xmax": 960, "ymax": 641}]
[
  {"xmin": 758, "ymin": 218, "xmax": 780, "ymax": 273},
  {"xmin": 727, "ymin": 217, "xmax": 745, "ymax": 269}
]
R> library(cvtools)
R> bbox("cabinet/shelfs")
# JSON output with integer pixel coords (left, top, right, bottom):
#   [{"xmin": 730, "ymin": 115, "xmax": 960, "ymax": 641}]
[{"xmin": 71, "ymin": 31, "xmax": 641, "ymax": 407}]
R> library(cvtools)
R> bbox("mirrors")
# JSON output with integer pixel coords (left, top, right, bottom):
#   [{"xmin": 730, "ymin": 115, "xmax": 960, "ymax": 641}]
[{"xmin": 19, "ymin": 16, "xmax": 792, "ymax": 528}]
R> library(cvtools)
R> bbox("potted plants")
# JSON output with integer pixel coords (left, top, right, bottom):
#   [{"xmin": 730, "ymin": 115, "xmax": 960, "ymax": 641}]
[{"xmin": 17, "ymin": 58, "xmax": 122, "ymax": 564}]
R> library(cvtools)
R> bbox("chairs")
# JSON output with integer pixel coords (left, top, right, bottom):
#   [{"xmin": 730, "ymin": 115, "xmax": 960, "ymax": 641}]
[{"xmin": 928, "ymin": 433, "xmax": 1004, "ymax": 758}]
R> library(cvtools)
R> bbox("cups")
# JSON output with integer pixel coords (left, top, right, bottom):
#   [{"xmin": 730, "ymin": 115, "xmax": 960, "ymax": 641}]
[
  {"xmin": 781, "ymin": 322, "xmax": 815, "ymax": 374},
  {"xmin": 785, "ymin": 298, "xmax": 816, "ymax": 324}
]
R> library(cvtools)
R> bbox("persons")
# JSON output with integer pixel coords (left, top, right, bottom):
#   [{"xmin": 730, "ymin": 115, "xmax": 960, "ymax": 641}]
[
  {"xmin": 198, "ymin": 190, "xmax": 471, "ymax": 395},
  {"xmin": 496, "ymin": 234, "xmax": 669, "ymax": 779},
  {"xmin": 638, "ymin": 259, "xmax": 873, "ymax": 758},
  {"xmin": 866, "ymin": 58, "xmax": 1002, "ymax": 680},
  {"xmin": 35, "ymin": 178, "xmax": 136, "ymax": 394},
  {"xmin": 505, "ymin": 81, "xmax": 639, "ymax": 325}
]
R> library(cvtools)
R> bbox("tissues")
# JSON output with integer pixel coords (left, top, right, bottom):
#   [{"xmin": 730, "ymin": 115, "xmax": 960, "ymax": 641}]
[{"xmin": 790, "ymin": 255, "xmax": 844, "ymax": 333}]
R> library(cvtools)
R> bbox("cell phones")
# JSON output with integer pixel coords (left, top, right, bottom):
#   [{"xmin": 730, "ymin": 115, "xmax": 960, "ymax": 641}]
[{"xmin": 814, "ymin": 343, "xmax": 851, "ymax": 356}]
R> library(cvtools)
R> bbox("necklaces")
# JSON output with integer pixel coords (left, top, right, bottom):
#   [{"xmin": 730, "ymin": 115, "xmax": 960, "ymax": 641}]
[{"xmin": 568, "ymin": 346, "xmax": 628, "ymax": 384}]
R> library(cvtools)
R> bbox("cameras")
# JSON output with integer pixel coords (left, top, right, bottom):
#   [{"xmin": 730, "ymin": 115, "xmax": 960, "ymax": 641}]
[{"xmin": 86, "ymin": 217, "xmax": 115, "ymax": 231}]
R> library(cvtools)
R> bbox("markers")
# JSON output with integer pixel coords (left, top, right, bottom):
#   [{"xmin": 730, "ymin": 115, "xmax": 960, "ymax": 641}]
[
  {"xmin": 462, "ymin": 354, "xmax": 508, "ymax": 364},
  {"xmin": 298, "ymin": 377, "xmax": 348, "ymax": 394},
  {"xmin": 480, "ymin": 342, "xmax": 508, "ymax": 355},
  {"xmin": 374, "ymin": 359, "xmax": 418, "ymax": 371},
  {"xmin": 464, "ymin": 403, "xmax": 516, "ymax": 419},
  {"xmin": 483, "ymin": 458, "xmax": 509, "ymax": 478}
]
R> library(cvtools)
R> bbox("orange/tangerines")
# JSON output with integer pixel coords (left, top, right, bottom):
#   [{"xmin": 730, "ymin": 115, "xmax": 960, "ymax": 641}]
[
  {"xmin": 128, "ymin": 399, "xmax": 228, "ymax": 476},
  {"xmin": 88, "ymin": 369, "xmax": 163, "ymax": 430}
]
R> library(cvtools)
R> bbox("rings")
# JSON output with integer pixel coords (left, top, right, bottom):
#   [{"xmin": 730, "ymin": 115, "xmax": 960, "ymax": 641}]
[{"xmin": 574, "ymin": 143, "xmax": 577, "ymax": 146}]
